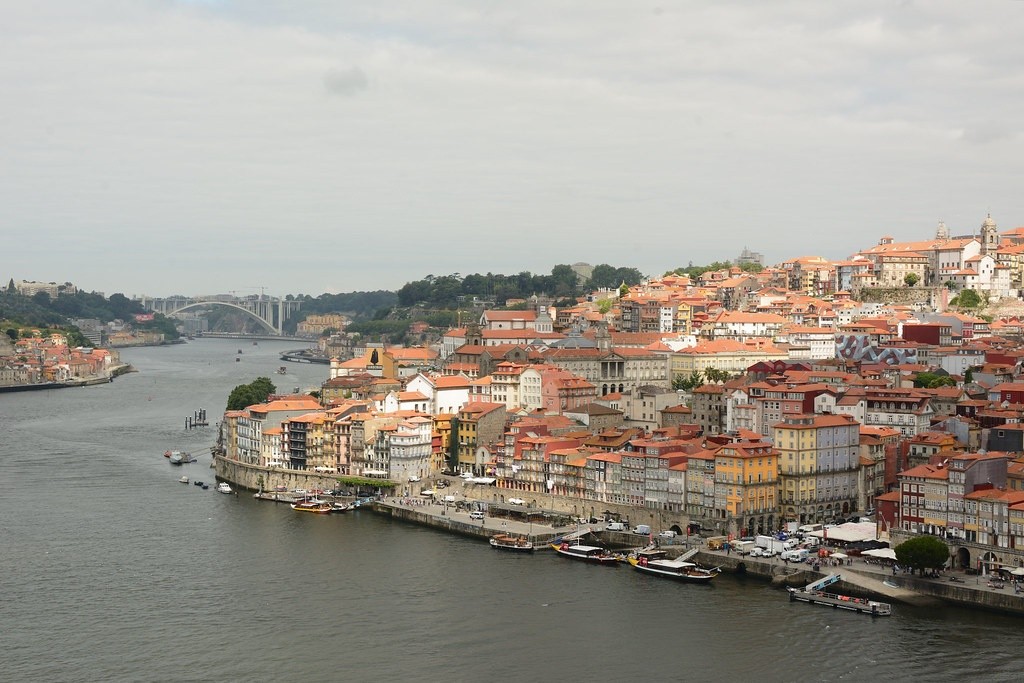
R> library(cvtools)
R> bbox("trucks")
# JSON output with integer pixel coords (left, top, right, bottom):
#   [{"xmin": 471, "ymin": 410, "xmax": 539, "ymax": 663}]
[
  {"xmin": 632, "ymin": 524, "xmax": 651, "ymax": 535},
  {"xmin": 606, "ymin": 522, "xmax": 624, "ymax": 531},
  {"xmin": 736, "ymin": 516, "xmax": 870, "ymax": 563}
]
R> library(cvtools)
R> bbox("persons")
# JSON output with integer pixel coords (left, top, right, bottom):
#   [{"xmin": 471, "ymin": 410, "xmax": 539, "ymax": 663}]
[
  {"xmin": 383, "ymin": 489, "xmax": 468, "ymax": 508},
  {"xmin": 592, "ymin": 539, "xmax": 655, "ymax": 558},
  {"xmin": 998, "ymin": 573, "xmax": 1022, "ymax": 594},
  {"xmin": 742, "ymin": 530, "xmax": 940, "ymax": 581}
]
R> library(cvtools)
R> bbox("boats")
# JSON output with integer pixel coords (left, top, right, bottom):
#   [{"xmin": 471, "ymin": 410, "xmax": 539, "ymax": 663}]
[
  {"xmin": 218, "ymin": 481, "xmax": 233, "ymax": 494},
  {"xmin": 626, "ymin": 555, "xmax": 720, "ymax": 582},
  {"xmin": 170, "ymin": 449, "xmax": 183, "ymax": 463},
  {"xmin": 550, "ymin": 520, "xmax": 623, "ymax": 563},
  {"xmin": 488, "ymin": 533, "xmax": 533, "ymax": 551},
  {"xmin": 788, "ymin": 572, "xmax": 892, "ymax": 616},
  {"xmin": 290, "ymin": 485, "xmax": 359, "ymax": 512}
]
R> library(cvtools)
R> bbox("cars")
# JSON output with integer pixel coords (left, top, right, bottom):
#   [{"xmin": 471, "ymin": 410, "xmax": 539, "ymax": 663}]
[
  {"xmin": 864, "ymin": 508, "xmax": 875, "ymax": 516},
  {"xmin": 295, "ymin": 487, "xmax": 371, "ymax": 497}
]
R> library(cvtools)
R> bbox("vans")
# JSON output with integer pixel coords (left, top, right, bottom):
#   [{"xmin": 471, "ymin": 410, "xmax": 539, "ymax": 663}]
[
  {"xmin": 470, "ymin": 511, "xmax": 485, "ymax": 519},
  {"xmin": 659, "ymin": 530, "xmax": 676, "ymax": 538}
]
